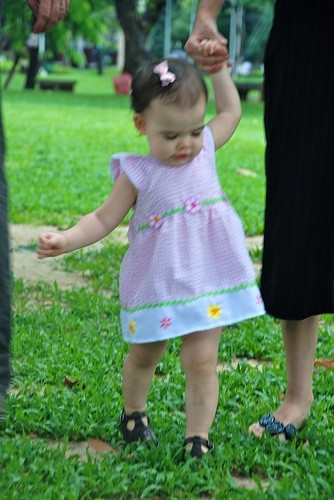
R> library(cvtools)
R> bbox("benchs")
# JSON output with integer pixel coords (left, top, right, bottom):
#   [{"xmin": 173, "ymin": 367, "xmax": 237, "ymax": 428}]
[
  {"xmin": 36, "ymin": 79, "xmax": 76, "ymax": 92},
  {"xmin": 234, "ymin": 82, "xmax": 263, "ymax": 102}
]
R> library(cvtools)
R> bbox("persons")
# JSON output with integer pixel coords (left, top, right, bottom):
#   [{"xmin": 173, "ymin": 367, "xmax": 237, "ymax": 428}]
[
  {"xmin": 37, "ymin": 40, "xmax": 266, "ymax": 465},
  {"xmin": 181, "ymin": 0, "xmax": 334, "ymax": 449},
  {"xmin": 24, "ymin": 13, "xmax": 45, "ymax": 90},
  {"xmin": 0, "ymin": 0, "xmax": 72, "ymax": 403}
]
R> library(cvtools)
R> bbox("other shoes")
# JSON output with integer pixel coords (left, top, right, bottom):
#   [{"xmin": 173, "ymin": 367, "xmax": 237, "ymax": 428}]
[
  {"xmin": 182, "ymin": 435, "xmax": 214, "ymax": 464},
  {"xmin": 119, "ymin": 408, "xmax": 158, "ymax": 448}
]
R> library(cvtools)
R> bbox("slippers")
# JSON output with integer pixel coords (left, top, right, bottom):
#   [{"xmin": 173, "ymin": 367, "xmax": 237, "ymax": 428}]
[{"xmin": 248, "ymin": 413, "xmax": 306, "ymax": 447}]
